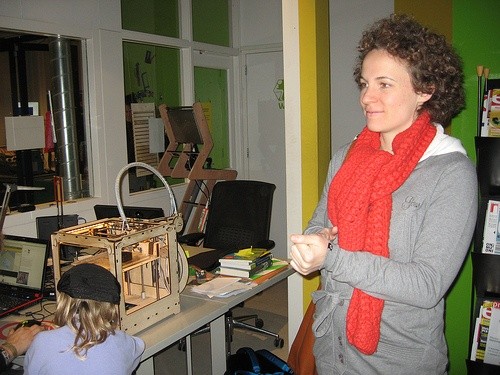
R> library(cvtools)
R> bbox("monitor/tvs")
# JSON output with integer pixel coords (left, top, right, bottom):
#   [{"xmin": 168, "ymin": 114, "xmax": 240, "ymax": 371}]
[{"xmin": 94, "ymin": 205, "xmax": 164, "ymax": 220}]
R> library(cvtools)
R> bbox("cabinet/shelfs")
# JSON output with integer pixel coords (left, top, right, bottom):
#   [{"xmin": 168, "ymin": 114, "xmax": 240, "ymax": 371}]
[{"xmin": 465, "ymin": 65, "xmax": 500, "ymax": 375}]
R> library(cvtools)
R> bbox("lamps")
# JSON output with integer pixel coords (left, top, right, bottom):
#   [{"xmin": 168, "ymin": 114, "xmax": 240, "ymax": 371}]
[{"xmin": 0, "ymin": 183, "xmax": 45, "ymax": 233}]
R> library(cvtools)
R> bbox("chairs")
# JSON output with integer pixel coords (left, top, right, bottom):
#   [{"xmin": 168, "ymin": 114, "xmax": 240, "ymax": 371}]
[{"xmin": 178, "ymin": 180, "xmax": 284, "ymax": 356}]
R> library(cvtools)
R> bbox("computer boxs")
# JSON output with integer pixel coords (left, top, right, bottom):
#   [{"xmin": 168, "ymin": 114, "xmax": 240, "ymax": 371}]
[{"xmin": 36, "ymin": 214, "xmax": 78, "ymax": 269}]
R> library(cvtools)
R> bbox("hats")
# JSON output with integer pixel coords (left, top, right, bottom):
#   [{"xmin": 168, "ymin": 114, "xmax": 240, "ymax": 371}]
[{"xmin": 57, "ymin": 263, "xmax": 121, "ymax": 305}]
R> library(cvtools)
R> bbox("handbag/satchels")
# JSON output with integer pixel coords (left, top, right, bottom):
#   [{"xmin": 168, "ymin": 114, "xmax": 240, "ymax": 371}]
[{"xmin": 224, "ymin": 347, "xmax": 294, "ymax": 375}]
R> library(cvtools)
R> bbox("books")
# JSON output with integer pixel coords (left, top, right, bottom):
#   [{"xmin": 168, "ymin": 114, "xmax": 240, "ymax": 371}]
[
  {"xmin": 481, "ymin": 89, "xmax": 500, "ymax": 137},
  {"xmin": 470, "ymin": 299, "xmax": 500, "ymax": 365},
  {"xmin": 215, "ymin": 248, "xmax": 274, "ymax": 278},
  {"xmin": 481, "ymin": 200, "xmax": 500, "ymax": 255}
]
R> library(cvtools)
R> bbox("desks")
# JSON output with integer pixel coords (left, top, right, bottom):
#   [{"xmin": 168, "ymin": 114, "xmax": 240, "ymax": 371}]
[{"xmin": 0, "ymin": 240, "xmax": 296, "ymax": 375}]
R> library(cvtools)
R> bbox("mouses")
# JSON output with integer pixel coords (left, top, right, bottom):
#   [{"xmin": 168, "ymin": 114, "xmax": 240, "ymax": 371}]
[{"xmin": 15, "ymin": 319, "xmax": 41, "ymax": 331}]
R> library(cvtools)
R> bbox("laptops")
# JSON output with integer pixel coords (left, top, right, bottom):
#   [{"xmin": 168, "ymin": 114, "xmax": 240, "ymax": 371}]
[{"xmin": 0, "ymin": 233, "xmax": 50, "ymax": 318}]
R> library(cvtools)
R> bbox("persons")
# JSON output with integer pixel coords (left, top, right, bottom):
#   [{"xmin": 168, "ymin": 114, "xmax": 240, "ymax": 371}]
[
  {"xmin": 0, "ymin": 325, "xmax": 43, "ymax": 369},
  {"xmin": 23, "ymin": 263, "xmax": 146, "ymax": 375},
  {"xmin": 124, "ymin": 93, "xmax": 147, "ymax": 193},
  {"xmin": 285, "ymin": 14, "xmax": 480, "ymax": 375}
]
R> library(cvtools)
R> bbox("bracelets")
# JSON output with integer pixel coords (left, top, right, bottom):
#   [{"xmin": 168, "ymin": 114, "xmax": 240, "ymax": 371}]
[{"xmin": 2, "ymin": 342, "xmax": 18, "ymax": 358}]
